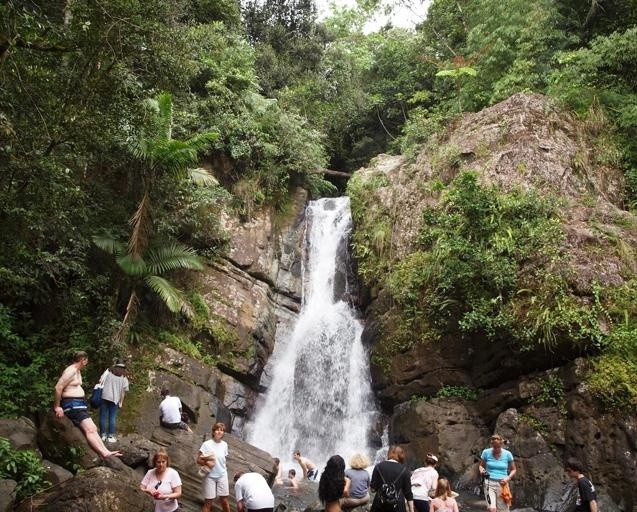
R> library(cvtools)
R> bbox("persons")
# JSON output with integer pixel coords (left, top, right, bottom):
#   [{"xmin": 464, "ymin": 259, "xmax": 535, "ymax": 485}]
[
  {"xmin": 234, "ymin": 471, "xmax": 274, "ymax": 512},
  {"xmin": 564, "ymin": 457, "xmax": 596, "ymax": 512},
  {"xmin": 53, "ymin": 351, "xmax": 123, "ymax": 461},
  {"xmin": 318, "ymin": 455, "xmax": 351, "ymax": 512},
  {"xmin": 411, "ymin": 453, "xmax": 438, "ymax": 512},
  {"xmin": 159, "ymin": 390, "xmax": 182, "ymax": 429},
  {"xmin": 429, "ymin": 479, "xmax": 457, "ymax": 512},
  {"xmin": 293, "ymin": 450, "xmax": 318, "ymax": 481},
  {"xmin": 140, "ymin": 449, "xmax": 182, "ymax": 512},
  {"xmin": 370, "ymin": 445, "xmax": 413, "ymax": 512},
  {"xmin": 197, "ymin": 422, "xmax": 231, "ymax": 512},
  {"xmin": 480, "ymin": 435, "xmax": 516, "ymax": 512},
  {"xmin": 92, "ymin": 358, "xmax": 129, "ymax": 443},
  {"xmin": 284, "ymin": 469, "xmax": 298, "ymax": 489},
  {"xmin": 340, "ymin": 453, "xmax": 370, "ymax": 508}
]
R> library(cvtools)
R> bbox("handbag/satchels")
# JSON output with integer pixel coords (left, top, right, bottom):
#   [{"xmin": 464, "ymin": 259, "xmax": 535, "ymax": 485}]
[{"xmin": 90, "ymin": 367, "xmax": 110, "ymax": 408}]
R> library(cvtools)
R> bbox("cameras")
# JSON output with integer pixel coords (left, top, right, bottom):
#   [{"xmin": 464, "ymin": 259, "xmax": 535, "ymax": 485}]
[{"xmin": 483, "ymin": 470, "xmax": 490, "ymax": 479}]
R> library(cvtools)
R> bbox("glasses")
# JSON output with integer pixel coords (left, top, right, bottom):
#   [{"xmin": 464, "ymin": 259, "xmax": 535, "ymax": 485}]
[{"xmin": 155, "ymin": 480, "xmax": 162, "ymax": 489}]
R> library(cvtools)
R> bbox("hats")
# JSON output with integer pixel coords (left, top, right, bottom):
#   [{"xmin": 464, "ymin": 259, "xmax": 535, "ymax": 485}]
[
  {"xmin": 115, "ymin": 358, "xmax": 126, "ymax": 368},
  {"xmin": 427, "ymin": 455, "xmax": 439, "ymax": 462}
]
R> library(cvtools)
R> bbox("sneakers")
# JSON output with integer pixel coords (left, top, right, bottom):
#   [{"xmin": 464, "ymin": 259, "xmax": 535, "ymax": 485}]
[
  {"xmin": 107, "ymin": 436, "xmax": 117, "ymax": 443},
  {"xmin": 101, "ymin": 435, "xmax": 107, "ymax": 441}
]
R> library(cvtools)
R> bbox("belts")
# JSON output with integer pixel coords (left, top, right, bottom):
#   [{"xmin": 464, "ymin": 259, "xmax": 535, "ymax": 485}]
[{"xmin": 63, "ymin": 406, "xmax": 88, "ymax": 412}]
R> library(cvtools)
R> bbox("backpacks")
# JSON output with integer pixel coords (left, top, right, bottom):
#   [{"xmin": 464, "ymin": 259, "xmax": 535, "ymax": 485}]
[{"xmin": 376, "ymin": 464, "xmax": 407, "ymax": 510}]
